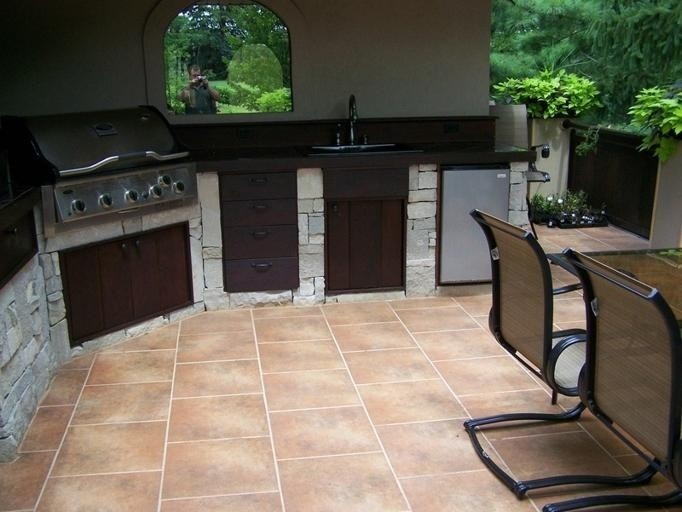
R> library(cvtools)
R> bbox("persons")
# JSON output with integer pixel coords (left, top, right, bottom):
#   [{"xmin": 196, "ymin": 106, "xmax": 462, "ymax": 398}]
[{"xmin": 174, "ymin": 63, "xmax": 219, "ymax": 114}]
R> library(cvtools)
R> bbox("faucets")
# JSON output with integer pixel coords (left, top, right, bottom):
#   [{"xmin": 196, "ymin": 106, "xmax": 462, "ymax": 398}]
[{"xmin": 348, "ymin": 94, "xmax": 359, "ymax": 146}]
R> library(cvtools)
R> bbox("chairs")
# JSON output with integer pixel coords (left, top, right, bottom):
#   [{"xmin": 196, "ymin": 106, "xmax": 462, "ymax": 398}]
[
  {"xmin": 536, "ymin": 249, "xmax": 681, "ymax": 511},
  {"xmin": 463, "ymin": 209, "xmax": 658, "ymax": 501}
]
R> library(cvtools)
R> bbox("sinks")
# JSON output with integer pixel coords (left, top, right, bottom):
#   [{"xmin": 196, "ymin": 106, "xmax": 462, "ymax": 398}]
[{"xmin": 307, "ymin": 143, "xmax": 425, "ymax": 157}]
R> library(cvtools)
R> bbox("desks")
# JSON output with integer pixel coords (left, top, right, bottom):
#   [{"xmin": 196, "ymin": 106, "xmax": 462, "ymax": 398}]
[{"xmin": 546, "ymin": 248, "xmax": 681, "ymax": 329}]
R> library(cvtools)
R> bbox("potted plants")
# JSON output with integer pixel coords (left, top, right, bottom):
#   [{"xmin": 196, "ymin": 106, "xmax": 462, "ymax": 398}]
[
  {"xmin": 490, "ymin": 66, "xmax": 603, "ymax": 118},
  {"xmin": 624, "ymin": 79, "xmax": 682, "ymax": 165}
]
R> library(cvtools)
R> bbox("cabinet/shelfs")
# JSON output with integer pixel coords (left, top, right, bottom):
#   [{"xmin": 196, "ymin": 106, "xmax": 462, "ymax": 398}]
[
  {"xmin": 0, "ymin": 189, "xmax": 41, "ymax": 289},
  {"xmin": 322, "ymin": 165, "xmax": 409, "ymax": 296},
  {"xmin": 216, "ymin": 167, "xmax": 301, "ymax": 294},
  {"xmin": 57, "ymin": 220, "xmax": 196, "ymax": 352}
]
